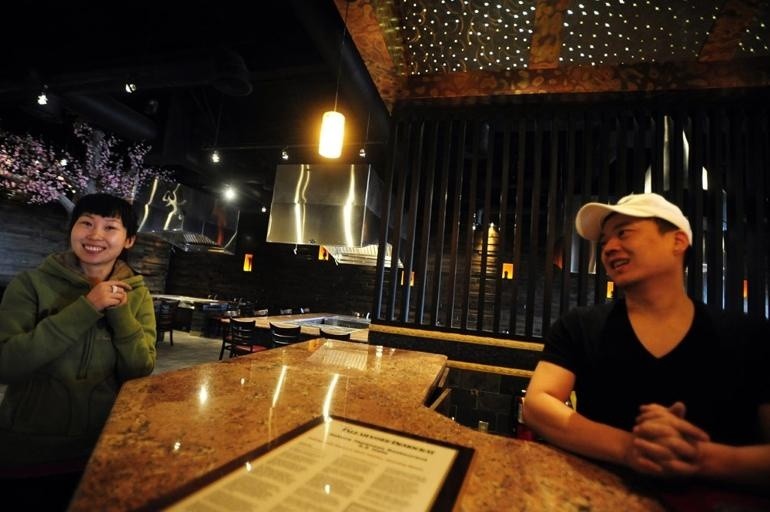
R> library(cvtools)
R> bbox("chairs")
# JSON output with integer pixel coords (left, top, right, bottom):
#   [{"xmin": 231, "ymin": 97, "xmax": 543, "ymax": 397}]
[{"xmin": 149, "ymin": 288, "xmax": 371, "ymax": 360}]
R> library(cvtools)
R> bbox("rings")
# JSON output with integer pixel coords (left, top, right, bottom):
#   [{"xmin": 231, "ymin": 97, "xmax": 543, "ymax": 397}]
[{"xmin": 111, "ymin": 284, "xmax": 119, "ymax": 294}]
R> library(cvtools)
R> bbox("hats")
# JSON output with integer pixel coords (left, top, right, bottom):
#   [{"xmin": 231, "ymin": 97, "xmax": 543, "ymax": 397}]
[{"xmin": 575, "ymin": 194, "xmax": 693, "ymax": 247}]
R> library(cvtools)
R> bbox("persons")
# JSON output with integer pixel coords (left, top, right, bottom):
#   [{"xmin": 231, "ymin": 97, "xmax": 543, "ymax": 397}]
[
  {"xmin": 518, "ymin": 186, "xmax": 770, "ymax": 512},
  {"xmin": 0, "ymin": 195, "xmax": 159, "ymax": 512}
]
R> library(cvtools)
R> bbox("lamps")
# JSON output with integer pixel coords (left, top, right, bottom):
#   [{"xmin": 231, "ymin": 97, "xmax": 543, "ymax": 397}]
[{"xmin": 318, "ymin": 2, "xmax": 350, "ymax": 160}]
